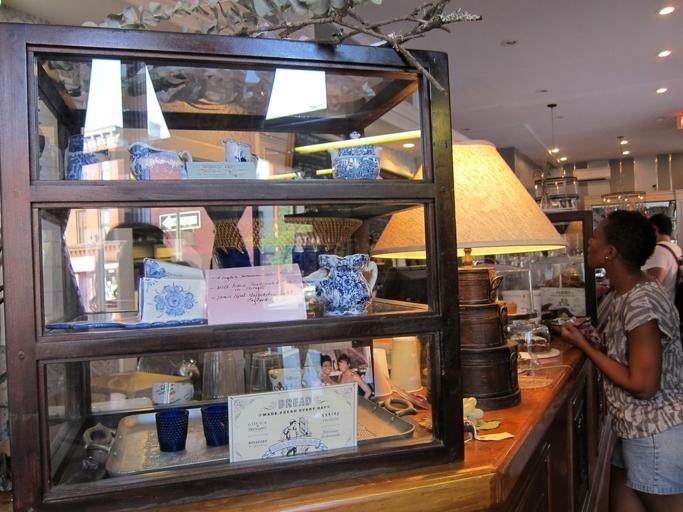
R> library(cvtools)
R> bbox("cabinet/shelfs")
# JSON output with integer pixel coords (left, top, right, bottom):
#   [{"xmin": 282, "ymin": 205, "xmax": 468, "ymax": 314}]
[
  {"xmin": 488, "ymin": 356, "xmax": 607, "ymax": 510},
  {"xmin": 0, "ymin": 23, "xmax": 467, "ymax": 511}
]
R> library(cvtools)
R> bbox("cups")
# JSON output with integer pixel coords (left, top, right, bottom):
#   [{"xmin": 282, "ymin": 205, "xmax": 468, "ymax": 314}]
[
  {"xmin": 200, "ymin": 404, "xmax": 229, "ymax": 447},
  {"xmin": 156, "ymin": 409, "xmax": 189, "ymax": 453}
]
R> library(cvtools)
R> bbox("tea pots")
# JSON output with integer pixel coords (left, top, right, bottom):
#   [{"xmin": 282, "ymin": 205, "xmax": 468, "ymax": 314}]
[
  {"xmin": 224, "ymin": 139, "xmax": 258, "ymax": 163},
  {"xmin": 126, "ymin": 140, "xmax": 194, "ymax": 182},
  {"xmin": 301, "ymin": 253, "xmax": 379, "ymax": 316},
  {"xmin": 331, "ymin": 131, "xmax": 380, "ymax": 181}
]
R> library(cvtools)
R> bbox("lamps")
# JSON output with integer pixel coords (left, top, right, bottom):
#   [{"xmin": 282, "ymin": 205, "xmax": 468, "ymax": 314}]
[
  {"xmin": 539, "ymin": 104, "xmax": 580, "ymax": 213},
  {"xmin": 373, "ymin": 140, "xmax": 566, "ymax": 411},
  {"xmin": 600, "ymin": 137, "xmax": 647, "ymax": 218}
]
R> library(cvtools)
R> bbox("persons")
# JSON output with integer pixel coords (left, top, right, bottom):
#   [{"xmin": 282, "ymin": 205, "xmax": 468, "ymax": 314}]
[
  {"xmin": 558, "ymin": 209, "xmax": 683, "ymax": 511},
  {"xmin": 283, "ymin": 421, "xmax": 298, "ymax": 456},
  {"xmin": 321, "ymin": 355, "xmax": 337, "ymax": 386},
  {"xmin": 337, "ymin": 353, "xmax": 372, "ymax": 400},
  {"xmin": 642, "ymin": 213, "xmax": 683, "ymax": 303}
]
